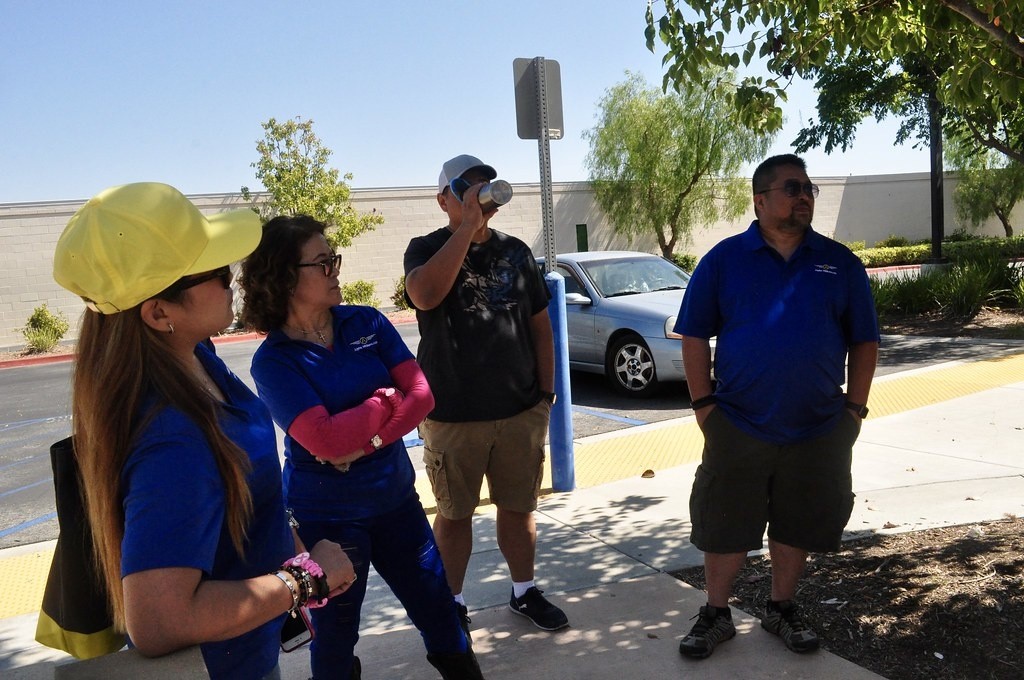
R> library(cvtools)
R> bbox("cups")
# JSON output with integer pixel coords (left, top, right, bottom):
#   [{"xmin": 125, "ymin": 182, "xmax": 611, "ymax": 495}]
[{"xmin": 477, "ymin": 180, "xmax": 513, "ymax": 214}]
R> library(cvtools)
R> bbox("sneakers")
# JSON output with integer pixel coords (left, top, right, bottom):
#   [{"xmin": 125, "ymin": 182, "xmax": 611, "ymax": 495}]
[
  {"xmin": 508, "ymin": 585, "xmax": 568, "ymax": 631},
  {"xmin": 679, "ymin": 605, "xmax": 736, "ymax": 659},
  {"xmin": 454, "ymin": 601, "xmax": 473, "ymax": 646},
  {"xmin": 760, "ymin": 600, "xmax": 820, "ymax": 653}
]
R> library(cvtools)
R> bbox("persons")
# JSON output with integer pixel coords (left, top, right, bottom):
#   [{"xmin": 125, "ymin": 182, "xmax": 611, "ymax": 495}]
[
  {"xmin": 241, "ymin": 213, "xmax": 485, "ymax": 680},
  {"xmin": 403, "ymin": 155, "xmax": 570, "ymax": 644},
  {"xmin": 673, "ymin": 154, "xmax": 880, "ymax": 662},
  {"xmin": 53, "ymin": 182, "xmax": 357, "ymax": 680}
]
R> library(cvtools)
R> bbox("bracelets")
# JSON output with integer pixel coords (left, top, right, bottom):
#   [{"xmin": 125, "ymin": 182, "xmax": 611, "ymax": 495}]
[
  {"xmin": 689, "ymin": 394, "xmax": 715, "ymax": 410},
  {"xmin": 268, "ymin": 551, "xmax": 330, "ymax": 618},
  {"xmin": 285, "ymin": 510, "xmax": 299, "ymax": 531},
  {"xmin": 374, "ymin": 388, "xmax": 399, "ymax": 416}
]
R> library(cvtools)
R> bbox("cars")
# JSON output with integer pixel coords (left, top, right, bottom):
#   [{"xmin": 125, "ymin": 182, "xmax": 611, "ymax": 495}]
[{"xmin": 534, "ymin": 251, "xmax": 718, "ymax": 403}]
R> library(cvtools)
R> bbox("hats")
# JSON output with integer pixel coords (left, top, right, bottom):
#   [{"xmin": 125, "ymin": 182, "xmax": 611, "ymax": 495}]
[
  {"xmin": 53, "ymin": 182, "xmax": 262, "ymax": 314},
  {"xmin": 438, "ymin": 155, "xmax": 497, "ymax": 194}
]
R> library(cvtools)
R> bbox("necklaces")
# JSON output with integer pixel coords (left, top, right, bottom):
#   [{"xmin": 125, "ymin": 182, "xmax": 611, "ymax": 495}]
[
  {"xmin": 155, "ymin": 336, "xmax": 215, "ymax": 392},
  {"xmin": 286, "ymin": 309, "xmax": 331, "ymax": 344}
]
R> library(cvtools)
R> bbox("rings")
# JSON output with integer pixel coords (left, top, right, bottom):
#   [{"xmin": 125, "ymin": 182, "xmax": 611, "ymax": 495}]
[
  {"xmin": 350, "ymin": 573, "xmax": 357, "ymax": 584},
  {"xmin": 319, "ymin": 458, "xmax": 326, "ymax": 464}
]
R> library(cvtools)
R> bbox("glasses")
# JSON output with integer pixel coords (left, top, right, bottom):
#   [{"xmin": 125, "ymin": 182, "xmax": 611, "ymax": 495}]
[
  {"xmin": 176, "ymin": 264, "xmax": 231, "ymax": 292},
  {"xmin": 756, "ymin": 183, "xmax": 820, "ymax": 198},
  {"xmin": 296, "ymin": 254, "xmax": 342, "ymax": 277}
]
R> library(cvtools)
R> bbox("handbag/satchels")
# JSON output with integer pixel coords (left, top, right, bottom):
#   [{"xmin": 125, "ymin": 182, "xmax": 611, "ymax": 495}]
[{"xmin": 35, "ymin": 434, "xmax": 132, "ymax": 660}]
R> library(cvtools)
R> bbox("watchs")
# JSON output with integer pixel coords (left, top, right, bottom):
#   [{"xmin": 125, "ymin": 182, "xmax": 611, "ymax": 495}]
[
  {"xmin": 847, "ymin": 400, "xmax": 868, "ymax": 418},
  {"xmin": 546, "ymin": 392, "xmax": 557, "ymax": 404},
  {"xmin": 370, "ymin": 435, "xmax": 382, "ymax": 449}
]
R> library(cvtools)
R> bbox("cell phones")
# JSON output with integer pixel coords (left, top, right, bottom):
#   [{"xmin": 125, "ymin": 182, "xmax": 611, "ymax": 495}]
[{"xmin": 280, "ymin": 607, "xmax": 315, "ymax": 653}]
